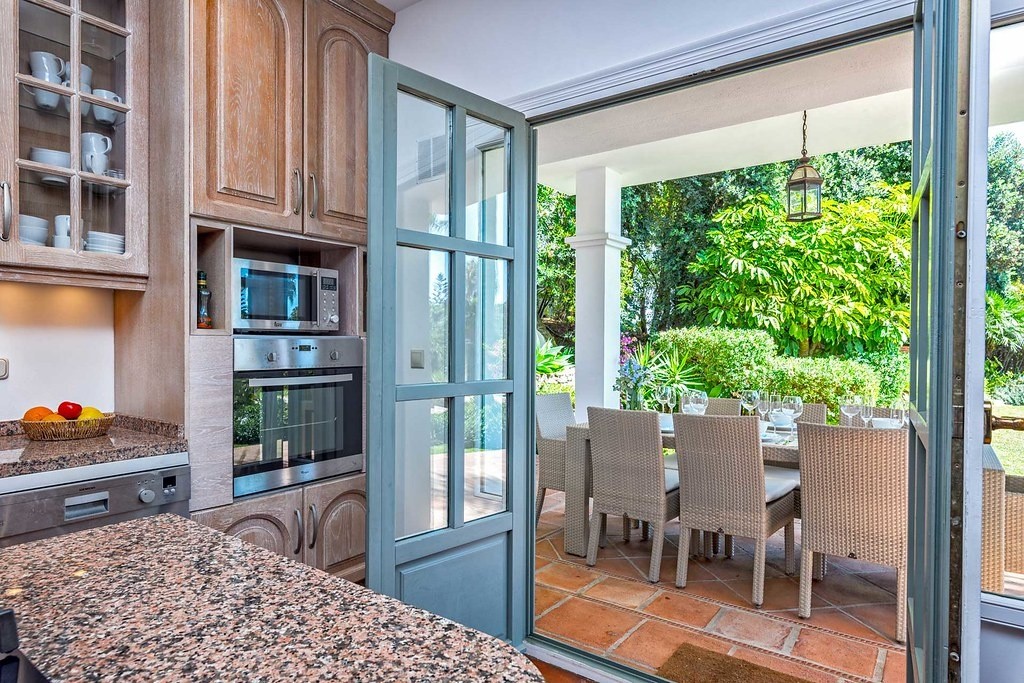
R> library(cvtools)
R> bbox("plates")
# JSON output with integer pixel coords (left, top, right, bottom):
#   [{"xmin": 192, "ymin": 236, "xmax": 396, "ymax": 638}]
[
  {"xmin": 661, "ymin": 429, "xmax": 674, "ymax": 433},
  {"xmin": 759, "ymin": 433, "xmax": 781, "ymax": 440},
  {"xmin": 766, "ymin": 423, "xmax": 797, "ymax": 431}
]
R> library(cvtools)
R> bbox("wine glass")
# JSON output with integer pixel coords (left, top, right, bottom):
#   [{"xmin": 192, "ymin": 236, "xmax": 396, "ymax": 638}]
[
  {"xmin": 742, "ymin": 390, "xmax": 803, "ymax": 441},
  {"xmin": 840, "ymin": 395, "xmax": 873, "ymax": 428},
  {"xmin": 656, "ymin": 386, "xmax": 677, "ymax": 416},
  {"xmin": 682, "ymin": 391, "xmax": 709, "ymax": 415}
]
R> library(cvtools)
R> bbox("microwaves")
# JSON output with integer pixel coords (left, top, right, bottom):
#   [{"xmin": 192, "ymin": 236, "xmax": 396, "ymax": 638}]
[{"xmin": 232, "ymin": 258, "xmax": 340, "ymax": 335}]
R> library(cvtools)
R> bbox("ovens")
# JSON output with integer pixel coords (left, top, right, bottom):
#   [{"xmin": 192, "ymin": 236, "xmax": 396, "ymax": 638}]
[{"xmin": 232, "ymin": 335, "xmax": 363, "ymax": 498}]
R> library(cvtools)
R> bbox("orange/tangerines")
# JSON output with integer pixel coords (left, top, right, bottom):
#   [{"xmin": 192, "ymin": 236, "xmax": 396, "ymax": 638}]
[{"xmin": 23, "ymin": 406, "xmax": 68, "ymax": 421}]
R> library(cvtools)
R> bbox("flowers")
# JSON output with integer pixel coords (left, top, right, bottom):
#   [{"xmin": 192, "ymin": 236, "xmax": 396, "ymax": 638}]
[{"xmin": 611, "ymin": 358, "xmax": 654, "ymax": 397}]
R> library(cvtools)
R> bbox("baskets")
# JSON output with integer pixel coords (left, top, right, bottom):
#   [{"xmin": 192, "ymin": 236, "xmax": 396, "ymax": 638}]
[{"xmin": 20, "ymin": 413, "xmax": 116, "ymax": 440}]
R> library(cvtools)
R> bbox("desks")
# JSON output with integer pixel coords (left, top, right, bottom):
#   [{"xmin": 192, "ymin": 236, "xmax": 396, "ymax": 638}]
[{"xmin": 564, "ymin": 422, "xmax": 1005, "ymax": 594}]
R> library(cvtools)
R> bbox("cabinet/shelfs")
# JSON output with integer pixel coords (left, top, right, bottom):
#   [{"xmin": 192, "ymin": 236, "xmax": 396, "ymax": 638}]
[
  {"xmin": 111, "ymin": 0, "xmax": 396, "ymax": 580},
  {"xmin": 0, "ymin": 0, "xmax": 149, "ymax": 292}
]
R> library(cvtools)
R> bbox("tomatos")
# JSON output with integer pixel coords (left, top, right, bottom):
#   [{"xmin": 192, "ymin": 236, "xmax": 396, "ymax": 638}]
[{"xmin": 58, "ymin": 401, "xmax": 83, "ymax": 419}]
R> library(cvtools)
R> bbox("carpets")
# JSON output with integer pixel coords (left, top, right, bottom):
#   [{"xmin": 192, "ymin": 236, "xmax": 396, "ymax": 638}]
[{"xmin": 654, "ymin": 642, "xmax": 814, "ymax": 683}]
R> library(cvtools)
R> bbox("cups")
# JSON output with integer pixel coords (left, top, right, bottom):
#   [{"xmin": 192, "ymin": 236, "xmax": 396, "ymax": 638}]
[
  {"xmin": 890, "ymin": 400, "xmax": 905, "ymax": 428},
  {"xmin": 22, "ymin": 51, "xmax": 123, "ymax": 124},
  {"xmin": 53, "ymin": 215, "xmax": 84, "ymax": 252},
  {"xmin": 79, "ymin": 132, "xmax": 112, "ymax": 185}
]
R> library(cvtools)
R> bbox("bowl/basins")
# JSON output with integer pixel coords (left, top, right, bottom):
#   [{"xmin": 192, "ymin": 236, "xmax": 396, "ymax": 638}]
[
  {"xmin": 30, "ymin": 147, "xmax": 70, "ymax": 185},
  {"xmin": 870, "ymin": 418, "xmax": 896, "ymax": 428},
  {"xmin": 18, "ymin": 214, "xmax": 49, "ymax": 246},
  {"xmin": 767, "ymin": 412, "xmax": 792, "ymax": 426},
  {"xmin": 85, "ymin": 230, "xmax": 124, "ymax": 256}
]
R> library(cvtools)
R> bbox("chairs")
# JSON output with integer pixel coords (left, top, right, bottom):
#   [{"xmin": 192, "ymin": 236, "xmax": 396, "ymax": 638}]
[{"xmin": 534, "ymin": 395, "xmax": 1024, "ymax": 644}]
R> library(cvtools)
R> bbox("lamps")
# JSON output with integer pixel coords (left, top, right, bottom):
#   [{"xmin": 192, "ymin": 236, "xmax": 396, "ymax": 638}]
[{"xmin": 786, "ymin": 109, "xmax": 823, "ymax": 222}]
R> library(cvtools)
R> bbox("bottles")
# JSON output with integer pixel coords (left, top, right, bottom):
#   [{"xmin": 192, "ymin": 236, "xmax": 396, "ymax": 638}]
[{"xmin": 196, "ymin": 271, "xmax": 212, "ymax": 329}]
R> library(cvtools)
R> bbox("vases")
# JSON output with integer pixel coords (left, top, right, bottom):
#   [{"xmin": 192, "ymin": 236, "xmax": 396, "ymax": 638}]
[{"xmin": 625, "ymin": 388, "xmax": 644, "ymax": 410}]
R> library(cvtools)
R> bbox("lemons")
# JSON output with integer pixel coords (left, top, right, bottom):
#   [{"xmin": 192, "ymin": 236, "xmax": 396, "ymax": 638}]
[{"xmin": 75, "ymin": 406, "xmax": 106, "ymax": 428}]
